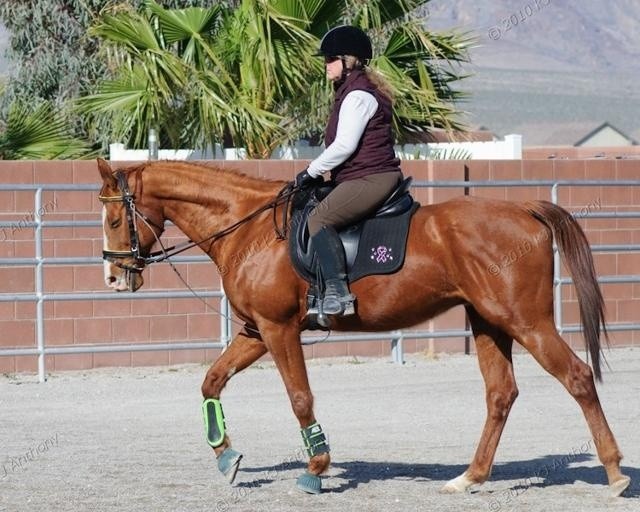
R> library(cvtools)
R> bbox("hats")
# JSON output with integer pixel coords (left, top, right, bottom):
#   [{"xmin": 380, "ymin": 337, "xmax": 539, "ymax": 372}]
[{"xmin": 310, "ymin": 25, "xmax": 373, "ymax": 59}]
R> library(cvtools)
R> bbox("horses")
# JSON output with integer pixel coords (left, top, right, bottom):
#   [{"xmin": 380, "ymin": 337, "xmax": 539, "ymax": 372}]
[{"xmin": 97, "ymin": 157, "xmax": 631, "ymax": 497}]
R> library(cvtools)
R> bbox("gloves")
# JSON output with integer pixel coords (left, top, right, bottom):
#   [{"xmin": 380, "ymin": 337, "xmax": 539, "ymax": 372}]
[{"xmin": 295, "ymin": 168, "xmax": 313, "ymax": 189}]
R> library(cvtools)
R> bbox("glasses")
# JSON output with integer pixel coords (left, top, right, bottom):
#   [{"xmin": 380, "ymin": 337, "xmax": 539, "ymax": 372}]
[{"xmin": 325, "ymin": 56, "xmax": 339, "ymax": 63}]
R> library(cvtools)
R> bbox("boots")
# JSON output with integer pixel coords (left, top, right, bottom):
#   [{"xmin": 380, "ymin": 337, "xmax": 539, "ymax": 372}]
[{"xmin": 303, "ymin": 223, "xmax": 357, "ymax": 320}]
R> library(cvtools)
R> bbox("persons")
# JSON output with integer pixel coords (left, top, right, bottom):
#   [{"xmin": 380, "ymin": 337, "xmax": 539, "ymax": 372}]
[{"xmin": 293, "ymin": 24, "xmax": 403, "ymax": 322}]
